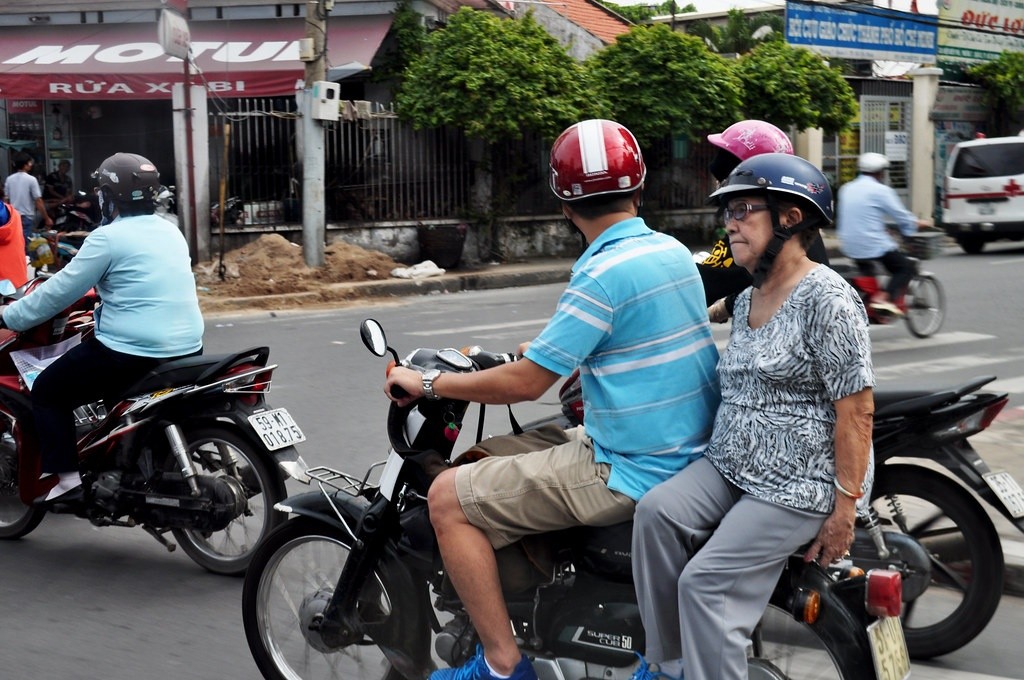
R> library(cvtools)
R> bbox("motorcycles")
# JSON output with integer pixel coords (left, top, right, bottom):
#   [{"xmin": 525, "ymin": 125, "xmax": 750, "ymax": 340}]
[
  {"xmin": 0, "ymin": 245, "xmax": 313, "ymax": 578},
  {"xmin": 242, "ymin": 318, "xmax": 913, "ymax": 680},
  {"xmin": 498, "ymin": 365, "xmax": 1024, "ymax": 664}
]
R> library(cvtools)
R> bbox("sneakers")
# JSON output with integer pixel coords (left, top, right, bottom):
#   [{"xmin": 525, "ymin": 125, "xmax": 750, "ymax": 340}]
[
  {"xmin": 426, "ymin": 643, "xmax": 540, "ymax": 680},
  {"xmin": 628, "ymin": 651, "xmax": 684, "ymax": 680}
]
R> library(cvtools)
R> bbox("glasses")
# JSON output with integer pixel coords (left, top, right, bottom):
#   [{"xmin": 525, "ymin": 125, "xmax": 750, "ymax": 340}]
[{"xmin": 721, "ymin": 199, "xmax": 787, "ymax": 225}]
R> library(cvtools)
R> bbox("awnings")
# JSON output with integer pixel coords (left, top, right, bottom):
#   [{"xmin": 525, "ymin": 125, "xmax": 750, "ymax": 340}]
[{"xmin": 0, "ymin": 13, "xmax": 398, "ymax": 108}]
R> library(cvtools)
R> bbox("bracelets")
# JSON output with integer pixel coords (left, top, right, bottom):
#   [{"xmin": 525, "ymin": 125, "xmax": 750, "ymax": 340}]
[{"xmin": 833, "ymin": 476, "xmax": 863, "ymax": 498}]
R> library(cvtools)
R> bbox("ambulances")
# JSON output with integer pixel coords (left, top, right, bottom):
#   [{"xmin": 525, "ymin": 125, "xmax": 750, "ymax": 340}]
[{"xmin": 940, "ymin": 131, "xmax": 1024, "ymax": 256}]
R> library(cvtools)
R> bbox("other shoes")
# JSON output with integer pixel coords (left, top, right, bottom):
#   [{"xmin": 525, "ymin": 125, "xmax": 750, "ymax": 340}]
[
  {"xmin": 31, "ymin": 483, "xmax": 90, "ymax": 508},
  {"xmin": 869, "ymin": 289, "xmax": 903, "ymax": 317}
]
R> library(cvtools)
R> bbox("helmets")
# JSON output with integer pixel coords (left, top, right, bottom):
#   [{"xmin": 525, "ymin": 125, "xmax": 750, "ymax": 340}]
[
  {"xmin": 548, "ymin": 117, "xmax": 647, "ymax": 202},
  {"xmin": 706, "ymin": 118, "xmax": 795, "ymax": 161},
  {"xmin": 90, "ymin": 152, "xmax": 160, "ymax": 208},
  {"xmin": 708, "ymin": 153, "xmax": 834, "ymax": 225}
]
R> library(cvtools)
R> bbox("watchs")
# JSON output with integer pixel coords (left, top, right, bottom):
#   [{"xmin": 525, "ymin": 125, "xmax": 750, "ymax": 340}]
[{"xmin": 422, "ymin": 369, "xmax": 442, "ymax": 399}]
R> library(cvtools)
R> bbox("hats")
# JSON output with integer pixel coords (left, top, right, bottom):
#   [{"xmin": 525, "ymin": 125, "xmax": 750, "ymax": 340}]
[{"xmin": 858, "ymin": 152, "xmax": 891, "ymax": 173}]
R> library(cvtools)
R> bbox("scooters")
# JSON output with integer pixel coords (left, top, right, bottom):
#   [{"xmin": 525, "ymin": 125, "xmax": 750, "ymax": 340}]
[
  {"xmin": 42, "ymin": 184, "xmax": 244, "ymax": 260},
  {"xmin": 826, "ymin": 226, "xmax": 946, "ymax": 340}
]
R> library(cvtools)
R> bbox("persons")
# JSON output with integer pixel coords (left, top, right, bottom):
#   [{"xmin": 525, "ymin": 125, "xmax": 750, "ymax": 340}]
[
  {"xmin": 0, "ymin": 152, "xmax": 204, "ymax": 506},
  {"xmin": 837, "ymin": 152, "xmax": 930, "ymax": 317},
  {"xmin": 386, "ymin": 119, "xmax": 875, "ymax": 680}
]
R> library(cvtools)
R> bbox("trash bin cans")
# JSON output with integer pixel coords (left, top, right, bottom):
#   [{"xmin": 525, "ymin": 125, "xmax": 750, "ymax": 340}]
[{"xmin": 417, "ymin": 223, "xmax": 467, "ymax": 270}]
